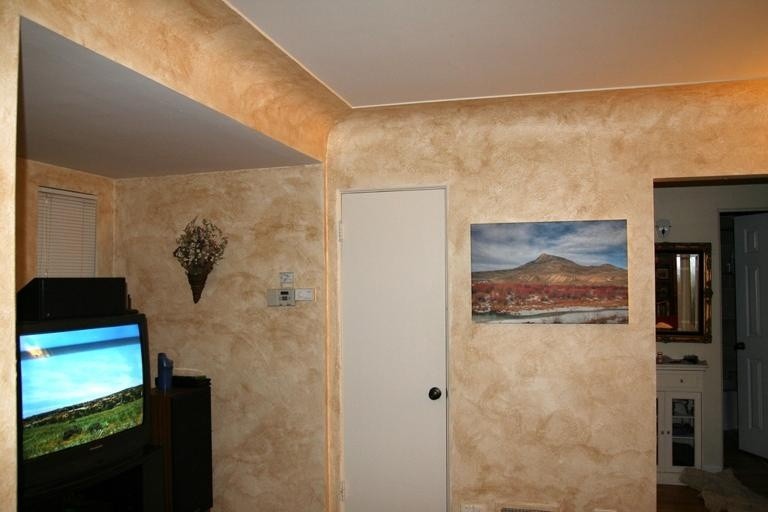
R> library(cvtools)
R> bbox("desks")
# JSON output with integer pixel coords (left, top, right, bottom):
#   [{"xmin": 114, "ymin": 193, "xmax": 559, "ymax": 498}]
[{"xmin": 17, "ymin": 445, "xmax": 167, "ymax": 512}]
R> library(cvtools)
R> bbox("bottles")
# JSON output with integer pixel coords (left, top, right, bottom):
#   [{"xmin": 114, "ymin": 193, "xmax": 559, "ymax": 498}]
[
  {"xmin": 657, "ymin": 352, "xmax": 663, "ymax": 364},
  {"xmin": 158, "ymin": 352, "xmax": 173, "ymax": 392}
]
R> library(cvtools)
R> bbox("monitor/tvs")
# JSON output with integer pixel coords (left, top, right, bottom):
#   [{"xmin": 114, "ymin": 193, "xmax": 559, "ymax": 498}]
[{"xmin": 16, "ymin": 313, "xmax": 154, "ymax": 489}]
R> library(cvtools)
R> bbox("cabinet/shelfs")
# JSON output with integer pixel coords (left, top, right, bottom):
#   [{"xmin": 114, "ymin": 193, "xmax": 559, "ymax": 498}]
[{"xmin": 657, "ymin": 364, "xmax": 710, "ymax": 487}]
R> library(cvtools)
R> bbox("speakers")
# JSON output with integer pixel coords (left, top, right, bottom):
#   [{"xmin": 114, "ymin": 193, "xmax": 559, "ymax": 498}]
[{"xmin": 16, "ymin": 277, "xmax": 126, "ymax": 320}]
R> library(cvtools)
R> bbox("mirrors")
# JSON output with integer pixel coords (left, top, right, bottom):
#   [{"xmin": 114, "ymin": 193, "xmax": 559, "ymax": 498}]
[{"xmin": 656, "ymin": 241, "xmax": 714, "ymax": 343}]
